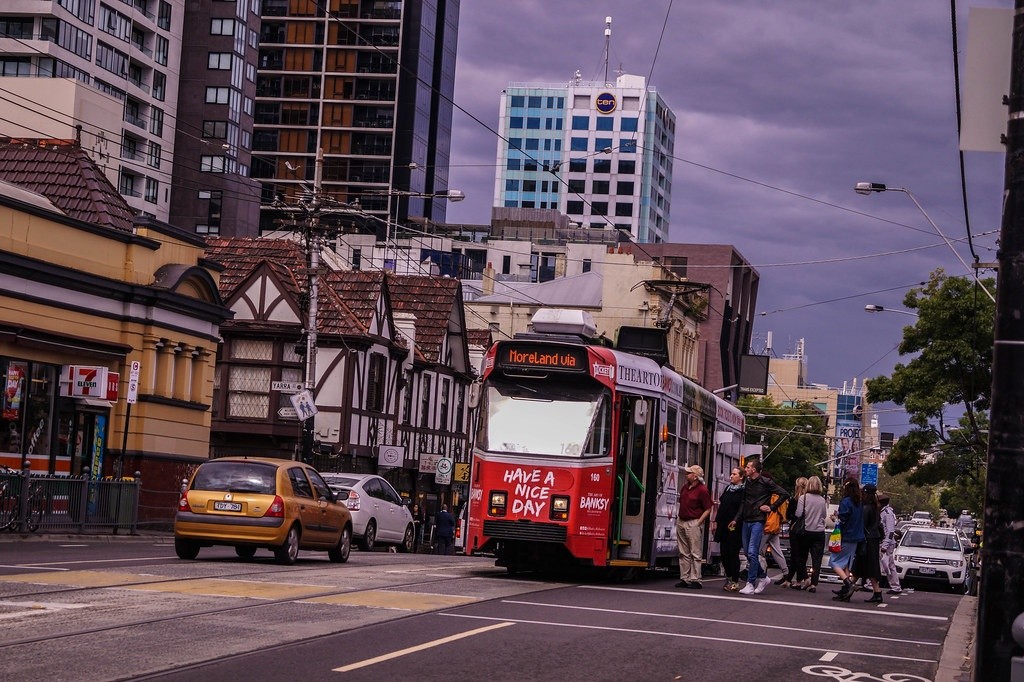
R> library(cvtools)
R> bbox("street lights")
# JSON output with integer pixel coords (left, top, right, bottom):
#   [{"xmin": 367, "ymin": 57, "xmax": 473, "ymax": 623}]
[{"xmin": 301, "ymin": 190, "xmax": 466, "ymax": 464}]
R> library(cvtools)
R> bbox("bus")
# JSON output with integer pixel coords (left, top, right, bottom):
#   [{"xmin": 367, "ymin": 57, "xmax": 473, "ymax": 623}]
[
  {"xmin": 909, "ymin": 511, "xmax": 932, "ymax": 522},
  {"xmin": 465, "ymin": 308, "xmax": 750, "ymax": 580},
  {"xmin": 896, "ymin": 513, "xmax": 909, "ymax": 520}
]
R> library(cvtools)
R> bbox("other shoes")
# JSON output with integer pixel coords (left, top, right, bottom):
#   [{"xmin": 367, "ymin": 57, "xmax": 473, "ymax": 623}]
[
  {"xmin": 801, "ymin": 580, "xmax": 812, "ymax": 590},
  {"xmin": 674, "ymin": 580, "xmax": 690, "ymax": 588},
  {"xmin": 774, "ymin": 574, "xmax": 789, "ymax": 585},
  {"xmin": 887, "ymin": 589, "xmax": 903, "ymax": 595},
  {"xmin": 781, "ymin": 579, "xmax": 792, "ymax": 588},
  {"xmin": 790, "ymin": 583, "xmax": 801, "ymax": 591},
  {"xmin": 809, "ymin": 587, "xmax": 816, "ymax": 593},
  {"xmin": 858, "ymin": 586, "xmax": 874, "ymax": 592},
  {"xmin": 686, "ymin": 581, "xmax": 702, "ymax": 589},
  {"xmin": 730, "ymin": 583, "xmax": 740, "ymax": 591},
  {"xmin": 723, "ymin": 579, "xmax": 732, "ymax": 591}
]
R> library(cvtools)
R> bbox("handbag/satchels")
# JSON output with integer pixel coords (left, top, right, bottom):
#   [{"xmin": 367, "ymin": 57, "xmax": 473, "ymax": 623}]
[
  {"xmin": 828, "ymin": 523, "xmax": 841, "ymax": 553},
  {"xmin": 879, "ymin": 552, "xmax": 891, "ymax": 576},
  {"xmin": 789, "ymin": 512, "xmax": 805, "ymax": 532}
]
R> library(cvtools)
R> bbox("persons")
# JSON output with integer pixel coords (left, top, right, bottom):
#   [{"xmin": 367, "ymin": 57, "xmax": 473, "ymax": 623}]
[
  {"xmin": 674, "ymin": 464, "xmax": 713, "ymax": 589},
  {"xmin": 435, "ymin": 504, "xmax": 456, "ymax": 554},
  {"xmin": 714, "ymin": 467, "xmax": 746, "ymax": 590},
  {"xmin": 728, "ymin": 458, "xmax": 790, "ymax": 595},
  {"xmin": 758, "ymin": 472, "xmax": 903, "ymax": 603},
  {"xmin": 836, "ymin": 438, "xmax": 861, "ymax": 484},
  {"xmin": 411, "ymin": 504, "xmax": 424, "ymax": 553}
]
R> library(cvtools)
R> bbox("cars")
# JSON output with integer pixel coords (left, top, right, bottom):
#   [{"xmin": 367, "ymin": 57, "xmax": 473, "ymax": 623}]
[
  {"xmin": 174, "ymin": 454, "xmax": 354, "ymax": 565},
  {"xmin": 765, "ymin": 524, "xmax": 791, "ymax": 567},
  {"xmin": 318, "ymin": 471, "xmax": 416, "ymax": 552}
]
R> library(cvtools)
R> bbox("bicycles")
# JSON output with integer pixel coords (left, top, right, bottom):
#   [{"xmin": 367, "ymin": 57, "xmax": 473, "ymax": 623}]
[{"xmin": 0, "ymin": 462, "xmax": 45, "ymax": 536}]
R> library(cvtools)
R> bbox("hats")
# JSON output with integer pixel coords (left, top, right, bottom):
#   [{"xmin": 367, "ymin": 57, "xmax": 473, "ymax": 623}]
[
  {"xmin": 861, "ymin": 484, "xmax": 876, "ymax": 495},
  {"xmin": 685, "ymin": 465, "xmax": 704, "ymax": 477},
  {"xmin": 877, "ymin": 495, "xmax": 890, "ymax": 507}
]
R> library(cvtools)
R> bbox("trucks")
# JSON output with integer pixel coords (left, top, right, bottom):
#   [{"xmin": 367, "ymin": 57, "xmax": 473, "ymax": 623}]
[
  {"xmin": 806, "ymin": 530, "xmax": 853, "ymax": 584},
  {"xmin": 957, "ymin": 514, "xmax": 977, "ymax": 527}
]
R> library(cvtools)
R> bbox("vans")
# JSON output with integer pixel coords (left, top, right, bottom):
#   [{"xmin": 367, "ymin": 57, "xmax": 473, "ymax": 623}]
[{"xmin": 454, "ymin": 502, "xmax": 468, "ymax": 556}]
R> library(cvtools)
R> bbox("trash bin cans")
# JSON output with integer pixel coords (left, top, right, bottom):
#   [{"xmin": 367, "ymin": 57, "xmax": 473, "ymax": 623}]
[{"xmin": 109, "ymin": 476, "xmax": 134, "ymax": 528}]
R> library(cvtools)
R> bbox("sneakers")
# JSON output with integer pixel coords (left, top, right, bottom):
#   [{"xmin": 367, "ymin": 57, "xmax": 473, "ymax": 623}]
[
  {"xmin": 738, "ymin": 582, "xmax": 754, "ymax": 595},
  {"xmin": 754, "ymin": 575, "xmax": 771, "ymax": 593}
]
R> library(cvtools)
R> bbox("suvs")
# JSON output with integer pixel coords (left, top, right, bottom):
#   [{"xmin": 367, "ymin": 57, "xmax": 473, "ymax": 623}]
[{"xmin": 892, "ymin": 521, "xmax": 980, "ymax": 594}]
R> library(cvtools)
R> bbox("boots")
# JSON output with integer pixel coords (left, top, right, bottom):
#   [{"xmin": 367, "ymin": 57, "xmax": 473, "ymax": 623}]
[
  {"xmin": 832, "ymin": 585, "xmax": 849, "ymax": 596},
  {"xmin": 844, "ymin": 577, "xmax": 855, "ymax": 595},
  {"xmin": 832, "ymin": 593, "xmax": 851, "ymax": 602},
  {"xmin": 864, "ymin": 591, "xmax": 883, "ymax": 604}
]
